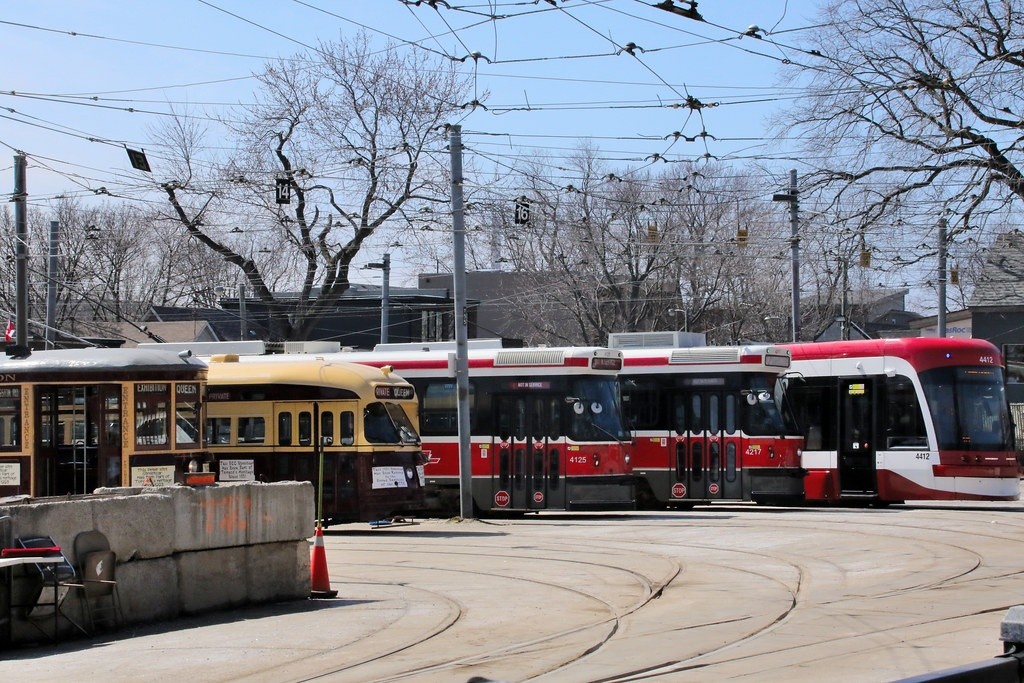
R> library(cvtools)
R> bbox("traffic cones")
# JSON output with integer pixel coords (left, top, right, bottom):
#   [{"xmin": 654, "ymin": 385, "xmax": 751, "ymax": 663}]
[{"xmin": 306, "ymin": 523, "xmax": 341, "ymax": 600}]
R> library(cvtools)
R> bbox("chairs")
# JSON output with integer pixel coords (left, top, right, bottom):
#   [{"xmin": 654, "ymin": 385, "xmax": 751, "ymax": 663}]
[
  {"xmin": 73, "ymin": 531, "xmax": 126, "ymax": 635},
  {"xmin": 17, "ymin": 535, "xmax": 118, "ymax": 646}
]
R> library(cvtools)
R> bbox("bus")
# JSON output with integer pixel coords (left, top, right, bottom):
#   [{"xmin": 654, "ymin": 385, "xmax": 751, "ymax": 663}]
[
  {"xmin": 0, "ymin": 346, "xmax": 425, "ymax": 519},
  {"xmin": 749, "ymin": 337, "xmax": 1024, "ymax": 509},
  {"xmin": 135, "ymin": 342, "xmax": 639, "ymax": 520},
  {"xmin": 507, "ymin": 331, "xmax": 811, "ymax": 513},
  {"xmin": 0, "ymin": 347, "xmax": 212, "ymax": 488}
]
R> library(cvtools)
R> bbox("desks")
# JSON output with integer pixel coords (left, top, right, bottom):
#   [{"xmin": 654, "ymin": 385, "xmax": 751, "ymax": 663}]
[{"xmin": 0, "ymin": 554, "xmax": 65, "ymax": 645}]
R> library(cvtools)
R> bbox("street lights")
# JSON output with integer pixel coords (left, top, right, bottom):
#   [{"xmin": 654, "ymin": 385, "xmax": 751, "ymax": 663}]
[
  {"xmin": 773, "ymin": 171, "xmax": 802, "ymax": 346},
  {"xmin": 360, "ymin": 253, "xmax": 393, "ymax": 347}
]
